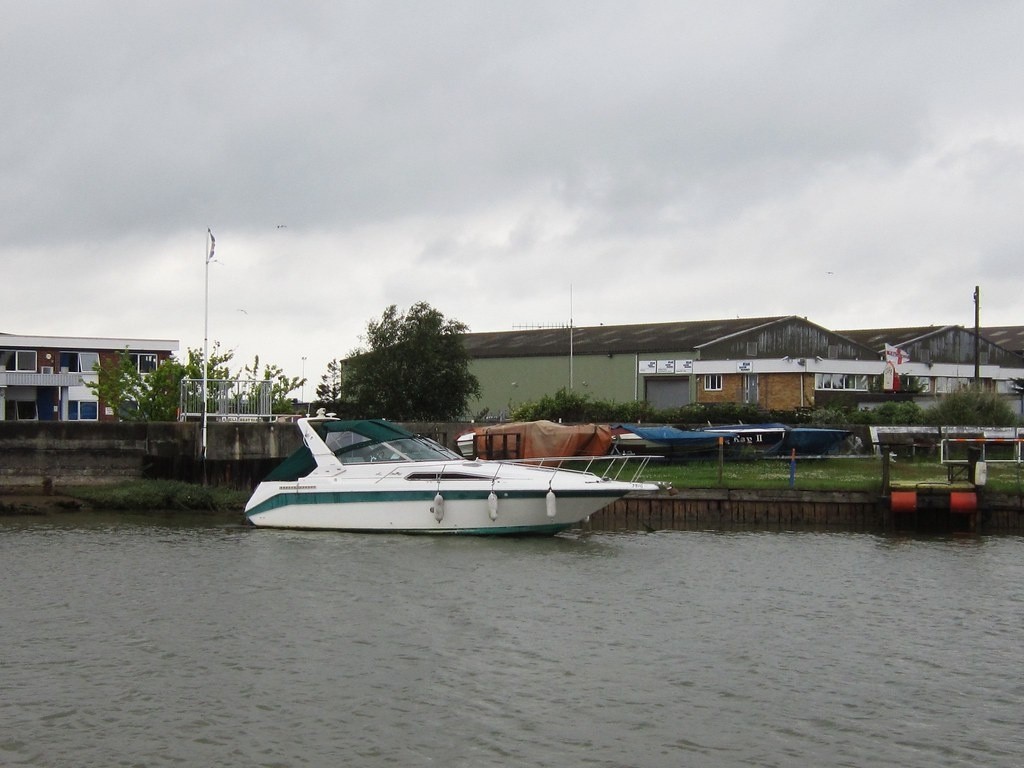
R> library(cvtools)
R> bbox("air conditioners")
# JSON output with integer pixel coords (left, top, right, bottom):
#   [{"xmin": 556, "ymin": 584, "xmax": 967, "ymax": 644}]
[{"xmin": 40, "ymin": 366, "xmax": 53, "ymax": 374}]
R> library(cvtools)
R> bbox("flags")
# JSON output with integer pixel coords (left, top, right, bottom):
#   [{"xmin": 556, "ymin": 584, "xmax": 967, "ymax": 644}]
[{"xmin": 883, "ymin": 343, "xmax": 912, "ymax": 366}]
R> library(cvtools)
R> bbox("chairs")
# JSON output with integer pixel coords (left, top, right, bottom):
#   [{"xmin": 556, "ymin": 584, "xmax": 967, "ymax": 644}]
[{"xmin": 943, "ymin": 447, "xmax": 980, "ymax": 483}]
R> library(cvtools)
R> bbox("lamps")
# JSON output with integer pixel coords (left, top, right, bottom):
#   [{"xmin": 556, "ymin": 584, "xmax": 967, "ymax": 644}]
[
  {"xmin": 781, "ymin": 356, "xmax": 793, "ymax": 362},
  {"xmin": 815, "ymin": 356, "xmax": 823, "ymax": 364}
]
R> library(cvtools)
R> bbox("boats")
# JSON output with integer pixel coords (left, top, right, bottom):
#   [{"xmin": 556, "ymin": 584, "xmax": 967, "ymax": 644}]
[
  {"xmin": 608, "ymin": 421, "xmax": 739, "ymax": 461},
  {"xmin": 701, "ymin": 423, "xmax": 853, "ymax": 459},
  {"xmin": 245, "ymin": 405, "xmax": 659, "ymax": 536},
  {"xmin": 455, "ymin": 420, "xmax": 612, "ymax": 466}
]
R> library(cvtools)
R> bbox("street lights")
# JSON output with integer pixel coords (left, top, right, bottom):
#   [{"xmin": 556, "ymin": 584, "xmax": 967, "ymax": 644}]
[{"xmin": 300, "ymin": 355, "xmax": 308, "ymax": 403}]
